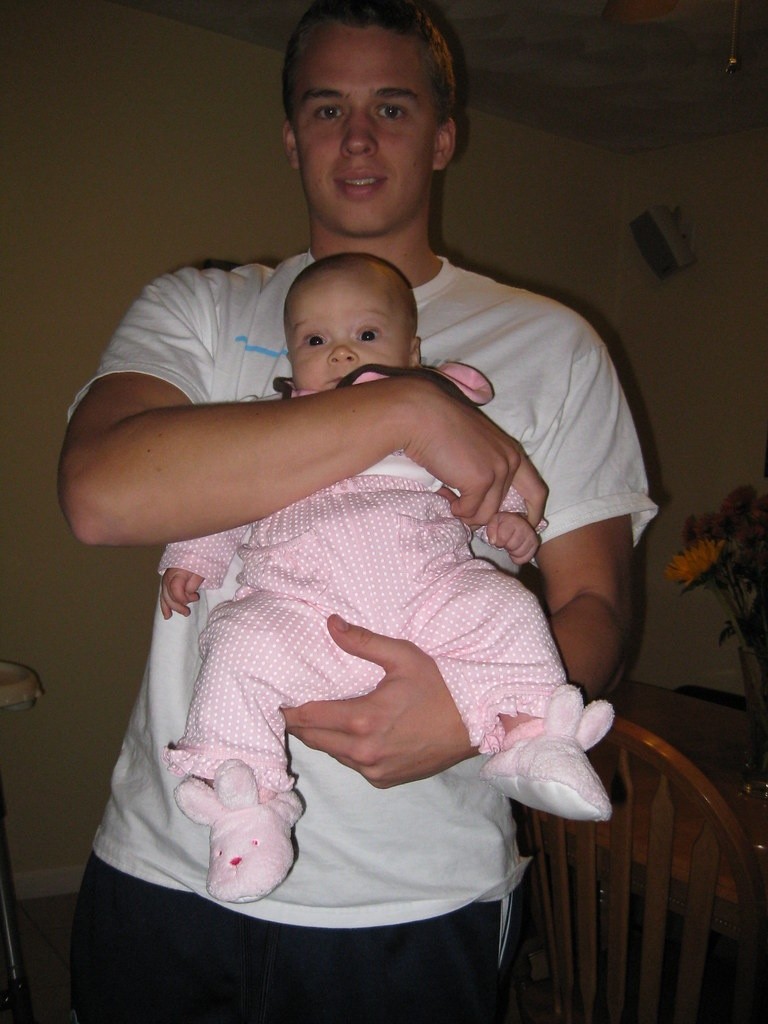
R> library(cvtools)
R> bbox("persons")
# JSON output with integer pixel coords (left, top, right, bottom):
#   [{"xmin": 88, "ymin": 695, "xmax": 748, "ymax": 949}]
[
  {"xmin": 57, "ymin": 0, "xmax": 655, "ymax": 1023},
  {"xmin": 158, "ymin": 252, "xmax": 615, "ymax": 904}
]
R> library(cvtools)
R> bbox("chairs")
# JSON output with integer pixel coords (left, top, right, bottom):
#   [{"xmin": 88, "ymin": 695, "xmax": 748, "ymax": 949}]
[{"xmin": 512, "ymin": 716, "xmax": 768, "ymax": 1023}]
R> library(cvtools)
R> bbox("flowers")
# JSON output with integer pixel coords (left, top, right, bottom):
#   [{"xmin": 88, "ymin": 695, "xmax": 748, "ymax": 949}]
[{"xmin": 663, "ymin": 481, "xmax": 768, "ymax": 648}]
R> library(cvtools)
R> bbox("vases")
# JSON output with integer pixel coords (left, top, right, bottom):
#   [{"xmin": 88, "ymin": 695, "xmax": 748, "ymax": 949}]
[{"xmin": 736, "ymin": 646, "xmax": 768, "ymax": 801}]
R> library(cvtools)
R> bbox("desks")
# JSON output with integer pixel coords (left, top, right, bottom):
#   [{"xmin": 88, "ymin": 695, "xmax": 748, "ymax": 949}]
[{"xmin": 512, "ymin": 680, "xmax": 768, "ymax": 964}]
